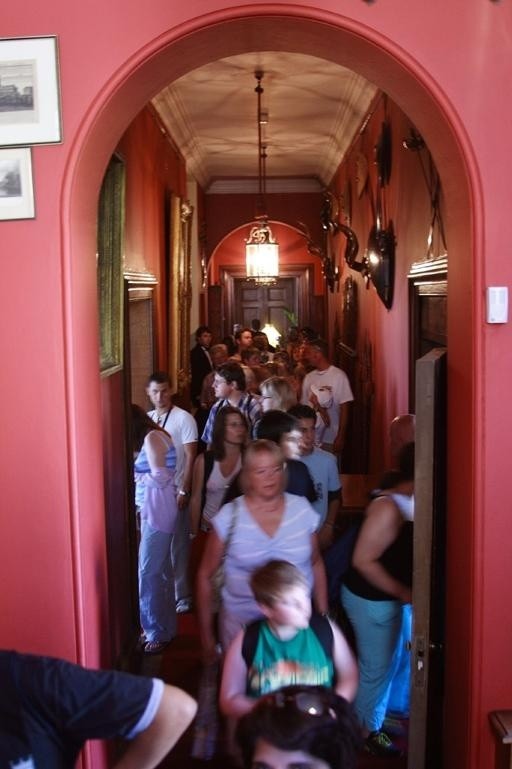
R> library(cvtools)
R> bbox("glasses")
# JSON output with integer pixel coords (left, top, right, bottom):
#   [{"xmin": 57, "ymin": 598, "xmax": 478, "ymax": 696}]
[{"xmin": 247, "ymin": 693, "xmax": 335, "ymax": 724}]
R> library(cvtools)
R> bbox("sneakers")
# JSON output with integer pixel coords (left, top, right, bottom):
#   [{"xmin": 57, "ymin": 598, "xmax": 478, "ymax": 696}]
[
  {"xmin": 383, "ymin": 720, "xmax": 402, "ymax": 735},
  {"xmin": 175, "ymin": 596, "xmax": 192, "ymax": 614},
  {"xmin": 365, "ymin": 731, "xmax": 403, "ymax": 757}
]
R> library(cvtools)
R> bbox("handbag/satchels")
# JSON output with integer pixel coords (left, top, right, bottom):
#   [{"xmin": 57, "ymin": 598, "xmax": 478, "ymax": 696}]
[{"xmin": 209, "ymin": 564, "xmax": 225, "ymax": 613}]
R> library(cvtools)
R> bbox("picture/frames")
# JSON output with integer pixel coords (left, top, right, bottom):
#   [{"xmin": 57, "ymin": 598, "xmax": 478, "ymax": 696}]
[
  {"xmin": 98, "ymin": 152, "xmax": 125, "ymax": 380},
  {"xmin": 0, "ymin": 35, "xmax": 63, "ymax": 147},
  {"xmin": 0, "ymin": 147, "xmax": 36, "ymax": 220},
  {"xmin": 168, "ymin": 192, "xmax": 193, "ymax": 396}
]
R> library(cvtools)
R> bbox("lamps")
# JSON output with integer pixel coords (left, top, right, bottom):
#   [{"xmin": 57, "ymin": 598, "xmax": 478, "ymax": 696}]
[{"xmin": 245, "ymin": 71, "xmax": 280, "ymax": 286}]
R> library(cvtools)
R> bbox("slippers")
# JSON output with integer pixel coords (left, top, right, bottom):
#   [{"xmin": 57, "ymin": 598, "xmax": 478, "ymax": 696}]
[{"xmin": 144, "ymin": 638, "xmax": 169, "ymax": 654}]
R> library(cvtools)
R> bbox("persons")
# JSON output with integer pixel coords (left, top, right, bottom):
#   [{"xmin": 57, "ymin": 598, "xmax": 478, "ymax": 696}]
[
  {"xmin": 335, "ymin": 442, "xmax": 413, "ymax": 761},
  {"xmin": 128, "ymin": 318, "xmax": 361, "ymax": 768},
  {"xmin": 0, "ymin": 648, "xmax": 200, "ymax": 768},
  {"xmin": 364, "ymin": 414, "xmax": 416, "ymax": 508}
]
